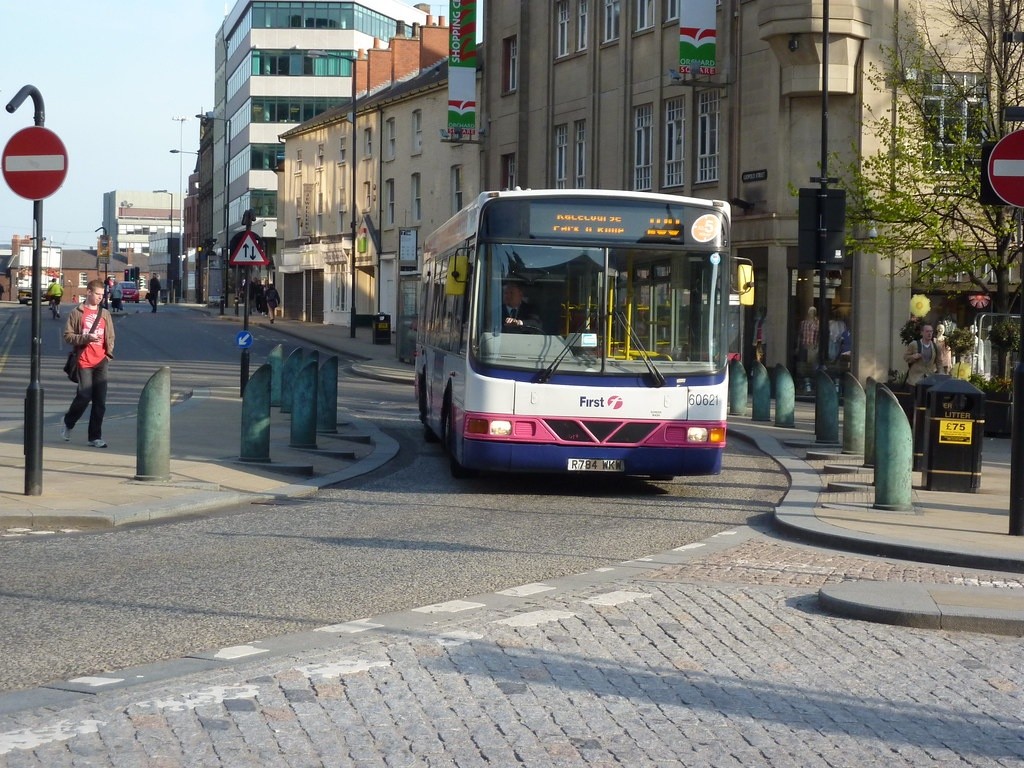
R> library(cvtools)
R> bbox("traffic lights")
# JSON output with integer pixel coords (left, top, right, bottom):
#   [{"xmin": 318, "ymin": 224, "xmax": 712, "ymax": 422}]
[
  {"xmin": 131, "ymin": 267, "xmax": 140, "ymax": 282},
  {"xmin": 124, "ymin": 269, "xmax": 129, "ymax": 281}
]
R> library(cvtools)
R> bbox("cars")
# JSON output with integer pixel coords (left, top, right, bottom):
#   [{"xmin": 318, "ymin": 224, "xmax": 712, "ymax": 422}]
[{"xmin": 118, "ymin": 282, "xmax": 140, "ymax": 303}]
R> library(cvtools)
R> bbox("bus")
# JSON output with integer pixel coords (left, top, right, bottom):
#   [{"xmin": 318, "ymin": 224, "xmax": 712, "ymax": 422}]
[{"xmin": 414, "ymin": 186, "xmax": 756, "ymax": 481}]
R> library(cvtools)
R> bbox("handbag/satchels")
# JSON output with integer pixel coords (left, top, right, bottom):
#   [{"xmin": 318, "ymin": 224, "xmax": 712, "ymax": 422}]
[
  {"xmin": 63, "ymin": 352, "xmax": 79, "ymax": 384},
  {"xmin": 145, "ymin": 292, "xmax": 150, "ymax": 299}
]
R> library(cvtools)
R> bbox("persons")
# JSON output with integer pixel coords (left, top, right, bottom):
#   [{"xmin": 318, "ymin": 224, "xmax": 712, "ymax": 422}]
[
  {"xmin": 149, "ymin": 273, "xmax": 161, "ymax": 313},
  {"xmin": 60, "ymin": 280, "xmax": 115, "ymax": 448},
  {"xmin": 904, "ymin": 321, "xmax": 943, "ymax": 416},
  {"xmin": 241, "ymin": 277, "xmax": 281, "ymax": 324},
  {"xmin": 934, "ymin": 314, "xmax": 992, "ymax": 374},
  {"xmin": 751, "ymin": 306, "xmax": 850, "ymax": 396},
  {"xmin": 493, "ymin": 281, "xmax": 544, "ymax": 335},
  {"xmin": 46, "ymin": 278, "xmax": 64, "ymax": 318},
  {"xmin": 72, "ymin": 277, "xmax": 123, "ymax": 312}
]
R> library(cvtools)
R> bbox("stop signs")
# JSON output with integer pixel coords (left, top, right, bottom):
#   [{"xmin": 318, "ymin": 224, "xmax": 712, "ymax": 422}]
[
  {"xmin": 2, "ymin": 127, "xmax": 70, "ymax": 203},
  {"xmin": 988, "ymin": 129, "xmax": 1024, "ymax": 209}
]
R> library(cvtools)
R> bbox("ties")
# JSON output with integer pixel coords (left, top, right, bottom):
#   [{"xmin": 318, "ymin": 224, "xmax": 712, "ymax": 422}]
[{"xmin": 512, "ymin": 308, "xmax": 517, "ymax": 320}]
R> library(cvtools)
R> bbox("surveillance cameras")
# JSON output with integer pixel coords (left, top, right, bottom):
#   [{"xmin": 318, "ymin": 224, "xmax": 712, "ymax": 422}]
[
  {"xmin": 789, "ymin": 40, "xmax": 797, "ymax": 53},
  {"xmin": 869, "ymin": 229, "xmax": 877, "ymax": 240},
  {"xmin": 263, "ymin": 223, "xmax": 266, "ymax": 226}
]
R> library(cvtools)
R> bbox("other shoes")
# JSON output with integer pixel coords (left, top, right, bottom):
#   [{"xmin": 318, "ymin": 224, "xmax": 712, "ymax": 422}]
[{"xmin": 271, "ymin": 319, "xmax": 274, "ymax": 324}]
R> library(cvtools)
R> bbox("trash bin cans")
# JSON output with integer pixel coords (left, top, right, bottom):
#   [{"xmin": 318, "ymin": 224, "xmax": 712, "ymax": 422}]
[
  {"xmin": 887, "ymin": 383, "xmax": 915, "ymax": 429},
  {"xmin": 371, "ymin": 312, "xmax": 392, "ymax": 345},
  {"xmin": 921, "ymin": 380, "xmax": 986, "ymax": 493},
  {"xmin": 911, "ymin": 374, "xmax": 952, "ymax": 473}
]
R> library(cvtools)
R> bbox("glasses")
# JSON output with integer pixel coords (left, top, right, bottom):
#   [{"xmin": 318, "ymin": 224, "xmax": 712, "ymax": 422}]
[{"xmin": 923, "ymin": 329, "xmax": 933, "ymax": 334}]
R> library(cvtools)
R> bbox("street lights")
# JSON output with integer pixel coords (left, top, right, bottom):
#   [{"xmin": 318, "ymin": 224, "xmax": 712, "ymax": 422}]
[
  {"xmin": 169, "ymin": 149, "xmax": 202, "ymax": 302},
  {"xmin": 196, "ymin": 114, "xmax": 231, "ymax": 308},
  {"xmin": 154, "ymin": 190, "xmax": 173, "ymax": 301},
  {"xmin": 307, "ymin": 51, "xmax": 357, "ymax": 339}
]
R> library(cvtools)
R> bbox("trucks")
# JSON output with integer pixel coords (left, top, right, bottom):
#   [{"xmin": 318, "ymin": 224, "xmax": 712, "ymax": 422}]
[{"xmin": 17, "ymin": 244, "xmax": 63, "ymax": 305}]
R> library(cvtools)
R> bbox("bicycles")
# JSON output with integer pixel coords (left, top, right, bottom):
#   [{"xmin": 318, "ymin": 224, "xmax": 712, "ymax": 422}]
[{"xmin": 50, "ymin": 299, "xmax": 58, "ymax": 319}]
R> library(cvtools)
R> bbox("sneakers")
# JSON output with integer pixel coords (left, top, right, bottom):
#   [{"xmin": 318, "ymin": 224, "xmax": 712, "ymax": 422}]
[
  {"xmin": 88, "ymin": 438, "xmax": 107, "ymax": 448},
  {"xmin": 61, "ymin": 414, "xmax": 72, "ymax": 441}
]
